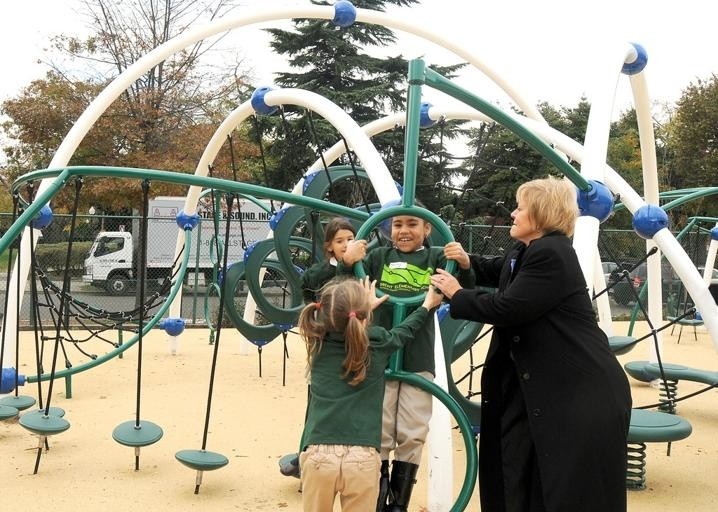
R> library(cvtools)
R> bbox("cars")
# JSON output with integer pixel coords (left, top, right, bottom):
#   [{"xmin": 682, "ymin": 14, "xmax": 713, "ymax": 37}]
[
  {"xmin": 695, "ymin": 267, "xmax": 717, "ymax": 285},
  {"xmin": 602, "ymin": 261, "xmax": 620, "ymax": 294}
]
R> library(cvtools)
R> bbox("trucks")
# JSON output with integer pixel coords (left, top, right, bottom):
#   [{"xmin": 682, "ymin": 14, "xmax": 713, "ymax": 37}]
[{"xmin": 79, "ymin": 195, "xmax": 282, "ymax": 296}]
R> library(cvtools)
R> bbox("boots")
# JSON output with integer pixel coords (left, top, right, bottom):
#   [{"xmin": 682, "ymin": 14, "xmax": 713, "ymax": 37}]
[
  {"xmin": 387, "ymin": 460, "xmax": 419, "ymax": 512},
  {"xmin": 376, "ymin": 459, "xmax": 390, "ymax": 512}
]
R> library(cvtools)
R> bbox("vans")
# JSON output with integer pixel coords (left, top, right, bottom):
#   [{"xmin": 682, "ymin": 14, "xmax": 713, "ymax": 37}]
[{"xmin": 606, "ymin": 257, "xmax": 683, "ymax": 307}]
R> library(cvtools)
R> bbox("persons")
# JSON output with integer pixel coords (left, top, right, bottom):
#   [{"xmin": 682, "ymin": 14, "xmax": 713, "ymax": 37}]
[
  {"xmin": 429, "ymin": 177, "xmax": 633, "ymax": 512},
  {"xmin": 335, "ymin": 214, "xmax": 477, "ymax": 512},
  {"xmin": 299, "ymin": 217, "xmax": 356, "ymax": 306},
  {"xmin": 298, "ymin": 275, "xmax": 444, "ymax": 512}
]
